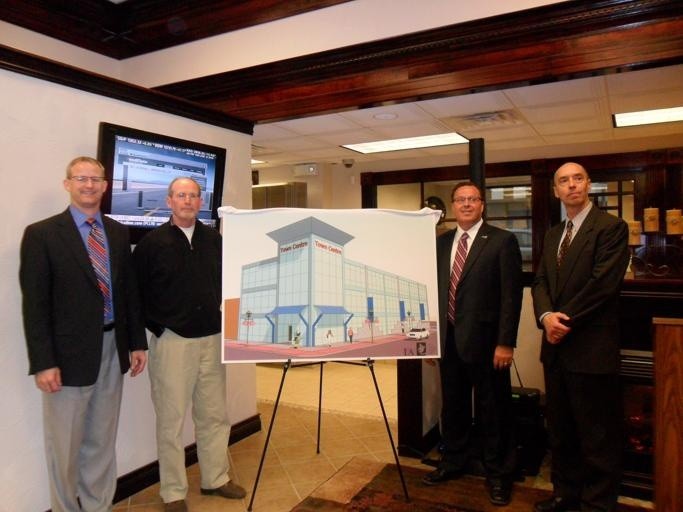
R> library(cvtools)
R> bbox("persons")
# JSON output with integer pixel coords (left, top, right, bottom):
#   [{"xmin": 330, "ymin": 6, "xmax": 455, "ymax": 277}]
[
  {"xmin": 347, "ymin": 326, "xmax": 354, "ymax": 344},
  {"xmin": 127, "ymin": 175, "xmax": 249, "ymax": 512},
  {"xmin": 325, "ymin": 328, "xmax": 334, "ymax": 348},
  {"xmin": 17, "ymin": 154, "xmax": 151, "ymax": 512},
  {"xmin": 289, "ymin": 331, "xmax": 304, "ymax": 349},
  {"xmin": 417, "ymin": 181, "xmax": 524, "ymax": 506},
  {"xmin": 528, "ymin": 160, "xmax": 631, "ymax": 511}
]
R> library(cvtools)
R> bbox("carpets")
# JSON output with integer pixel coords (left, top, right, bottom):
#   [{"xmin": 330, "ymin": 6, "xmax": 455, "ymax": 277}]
[{"xmin": 285, "ymin": 454, "xmax": 655, "ymax": 512}]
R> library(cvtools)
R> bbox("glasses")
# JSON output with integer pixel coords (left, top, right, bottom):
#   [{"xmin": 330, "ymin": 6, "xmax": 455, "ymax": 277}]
[
  {"xmin": 72, "ymin": 176, "xmax": 105, "ymax": 182},
  {"xmin": 452, "ymin": 197, "xmax": 483, "ymax": 202},
  {"xmin": 178, "ymin": 193, "xmax": 199, "ymax": 199}
]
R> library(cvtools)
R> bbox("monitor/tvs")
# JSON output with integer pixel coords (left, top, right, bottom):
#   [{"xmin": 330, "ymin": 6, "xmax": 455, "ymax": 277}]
[{"xmin": 96, "ymin": 121, "xmax": 226, "ymax": 245}]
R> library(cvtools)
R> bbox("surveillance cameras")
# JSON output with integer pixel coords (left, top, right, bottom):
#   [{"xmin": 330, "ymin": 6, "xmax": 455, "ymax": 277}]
[{"xmin": 343, "ymin": 159, "xmax": 354, "ymax": 168}]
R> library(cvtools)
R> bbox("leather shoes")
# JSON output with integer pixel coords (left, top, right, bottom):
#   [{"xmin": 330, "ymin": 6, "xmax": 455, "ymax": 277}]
[
  {"xmin": 486, "ymin": 476, "xmax": 512, "ymax": 504},
  {"xmin": 201, "ymin": 482, "xmax": 246, "ymax": 498},
  {"xmin": 165, "ymin": 500, "xmax": 187, "ymax": 512},
  {"xmin": 535, "ymin": 497, "xmax": 571, "ymax": 512},
  {"xmin": 422, "ymin": 469, "xmax": 461, "ymax": 485}
]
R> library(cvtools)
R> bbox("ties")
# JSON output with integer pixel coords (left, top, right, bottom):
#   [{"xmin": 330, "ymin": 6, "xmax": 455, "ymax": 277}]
[
  {"xmin": 448, "ymin": 233, "xmax": 469, "ymax": 321},
  {"xmin": 85, "ymin": 218, "xmax": 112, "ymax": 320},
  {"xmin": 556, "ymin": 222, "xmax": 573, "ymax": 268}
]
